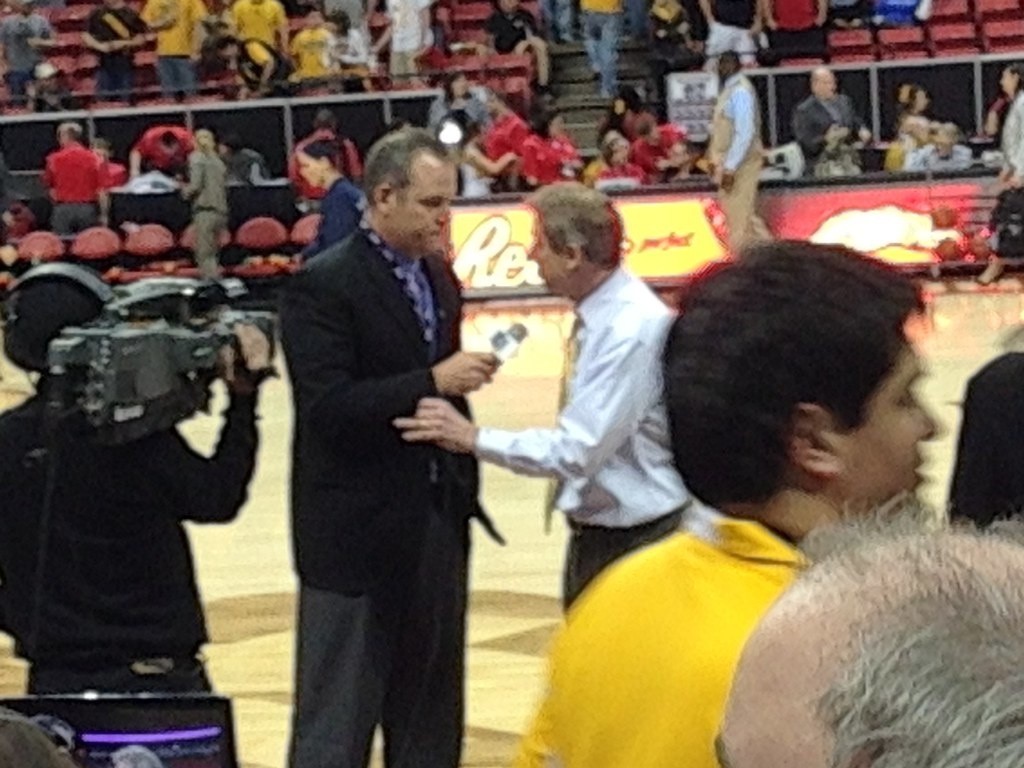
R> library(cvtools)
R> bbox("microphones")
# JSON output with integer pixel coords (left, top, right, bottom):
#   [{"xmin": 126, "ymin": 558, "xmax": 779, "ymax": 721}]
[
  {"xmin": 488, "ymin": 323, "xmax": 527, "ymax": 371},
  {"xmin": 200, "ymin": 270, "xmax": 263, "ymax": 309}
]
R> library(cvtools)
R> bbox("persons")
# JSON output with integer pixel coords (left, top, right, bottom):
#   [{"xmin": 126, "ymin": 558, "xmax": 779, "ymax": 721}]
[
  {"xmin": 975, "ymin": 64, "xmax": 1023, "ymax": 288},
  {"xmin": 1, "ymin": 256, "xmax": 273, "ymax": 697},
  {"xmin": 702, "ymin": 492, "xmax": 1024, "ymax": 768},
  {"xmin": 43, "ymin": 115, "xmax": 109, "ymax": 235},
  {"xmin": 696, "ymin": 49, "xmax": 778, "ymax": 260},
  {"xmin": 0, "ymin": 0, "xmax": 1024, "ymax": 212},
  {"xmin": 515, "ymin": 236, "xmax": 942, "ymax": 768},
  {"xmin": 181, "ymin": 126, "xmax": 230, "ymax": 284},
  {"xmin": 390, "ymin": 181, "xmax": 694, "ymax": 629},
  {"xmin": 279, "ymin": 127, "xmax": 505, "ymax": 768}
]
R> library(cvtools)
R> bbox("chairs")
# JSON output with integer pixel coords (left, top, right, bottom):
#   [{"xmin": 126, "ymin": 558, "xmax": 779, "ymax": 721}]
[{"xmin": 1, "ymin": 0, "xmax": 1023, "ymax": 299}]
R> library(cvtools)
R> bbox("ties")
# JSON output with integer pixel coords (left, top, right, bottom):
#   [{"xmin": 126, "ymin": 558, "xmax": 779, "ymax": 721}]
[{"xmin": 404, "ymin": 268, "xmax": 430, "ymax": 345}]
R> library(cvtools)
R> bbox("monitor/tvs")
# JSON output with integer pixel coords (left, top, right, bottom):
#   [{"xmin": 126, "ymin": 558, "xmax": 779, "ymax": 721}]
[{"xmin": 0, "ymin": 697, "xmax": 234, "ymax": 768}]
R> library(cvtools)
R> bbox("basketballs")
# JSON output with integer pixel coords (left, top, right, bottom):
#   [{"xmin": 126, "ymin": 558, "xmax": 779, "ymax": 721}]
[
  {"xmin": 968, "ymin": 231, "xmax": 993, "ymax": 261},
  {"xmin": 932, "ymin": 201, "xmax": 959, "ymax": 232},
  {"xmin": 936, "ymin": 238, "xmax": 961, "ymax": 264}
]
